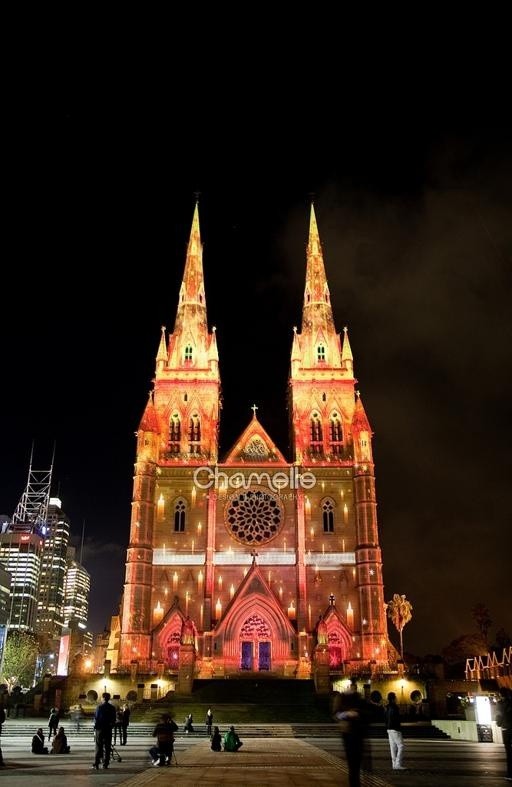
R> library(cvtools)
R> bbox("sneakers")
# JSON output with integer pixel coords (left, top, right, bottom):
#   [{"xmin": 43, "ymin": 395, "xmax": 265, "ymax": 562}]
[
  {"xmin": 165, "ymin": 757, "xmax": 169, "ymax": 762},
  {"xmin": 395, "ymin": 767, "xmax": 407, "ymax": 769},
  {"xmin": 153, "ymin": 758, "xmax": 160, "ymax": 765},
  {"xmin": 92, "ymin": 764, "xmax": 107, "ymax": 770}
]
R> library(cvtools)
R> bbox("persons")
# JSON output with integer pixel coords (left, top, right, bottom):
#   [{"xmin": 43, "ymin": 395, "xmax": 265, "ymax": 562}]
[
  {"xmin": 48, "ymin": 707, "xmax": 59, "ymax": 741},
  {"xmin": 183, "ymin": 713, "xmax": 194, "ymax": 734},
  {"xmin": 495, "ymin": 686, "xmax": 512, "ymax": 783},
  {"xmin": 223, "ymin": 726, "xmax": 243, "ymax": 752},
  {"xmin": 31, "ymin": 728, "xmax": 49, "ymax": 754},
  {"xmin": 205, "ymin": 708, "xmax": 214, "ymax": 735},
  {"xmin": 71, "ymin": 692, "xmax": 131, "ymax": 771},
  {"xmin": 210, "ymin": 728, "xmax": 222, "ymax": 751},
  {"xmin": 0, "ymin": 685, "xmax": 35, "ymax": 770},
  {"xmin": 329, "ymin": 683, "xmax": 407, "ymax": 787},
  {"xmin": 148, "ymin": 714, "xmax": 179, "ymax": 766},
  {"xmin": 50, "ymin": 727, "xmax": 70, "ymax": 754}
]
